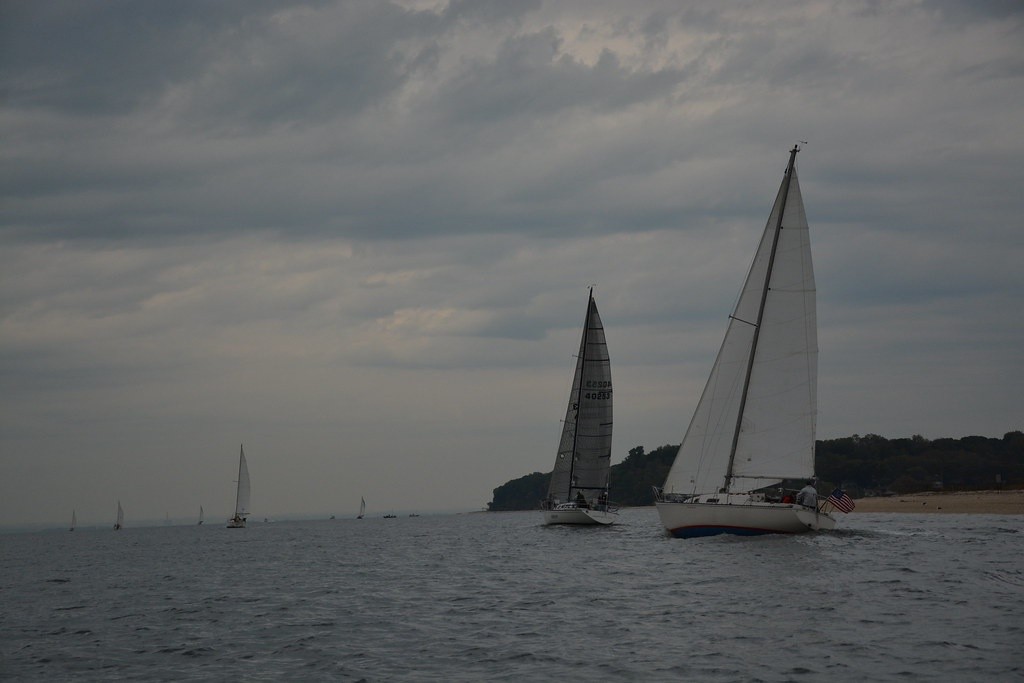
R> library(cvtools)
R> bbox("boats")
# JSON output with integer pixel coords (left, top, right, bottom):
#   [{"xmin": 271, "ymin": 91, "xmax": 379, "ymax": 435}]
[
  {"xmin": 383, "ymin": 515, "xmax": 396, "ymax": 518},
  {"xmin": 410, "ymin": 513, "xmax": 420, "ymax": 517}
]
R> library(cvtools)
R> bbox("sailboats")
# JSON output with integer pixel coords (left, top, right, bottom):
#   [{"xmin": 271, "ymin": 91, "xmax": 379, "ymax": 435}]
[
  {"xmin": 224, "ymin": 443, "xmax": 255, "ymax": 531},
  {"xmin": 649, "ymin": 142, "xmax": 859, "ymax": 534},
  {"xmin": 356, "ymin": 498, "xmax": 366, "ymax": 518},
  {"xmin": 69, "ymin": 510, "xmax": 76, "ymax": 532},
  {"xmin": 197, "ymin": 506, "xmax": 204, "ymax": 526},
  {"xmin": 540, "ymin": 286, "xmax": 623, "ymax": 527},
  {"xmin": 113, "ymin": 500, "xmax": 122, "ymax": 530}
]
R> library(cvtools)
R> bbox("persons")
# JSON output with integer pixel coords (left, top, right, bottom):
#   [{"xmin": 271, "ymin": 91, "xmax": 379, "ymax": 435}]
[
  {"xmin": 577, "ymin": 492, "xmax": 587, "ymax": 508},
  {"xmin": 782, "ymin": 490, "xmax": 796, "ymax": 503},
  {"xmin": 796, "ymin": 480, "xmax": 817, "ymax": 510},
  {"xmin": 234, "ymin": 516, "xmax": 240, "ymax": 522},
  {"xmin": 598, "ymin": 492, "xmax": 608, "ymax": 512}
]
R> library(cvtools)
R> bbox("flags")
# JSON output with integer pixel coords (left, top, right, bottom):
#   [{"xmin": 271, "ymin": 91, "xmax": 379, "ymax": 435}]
[{"xmin": 827, "ymin": 488, "xmax": 855, "ymax": 514}]
